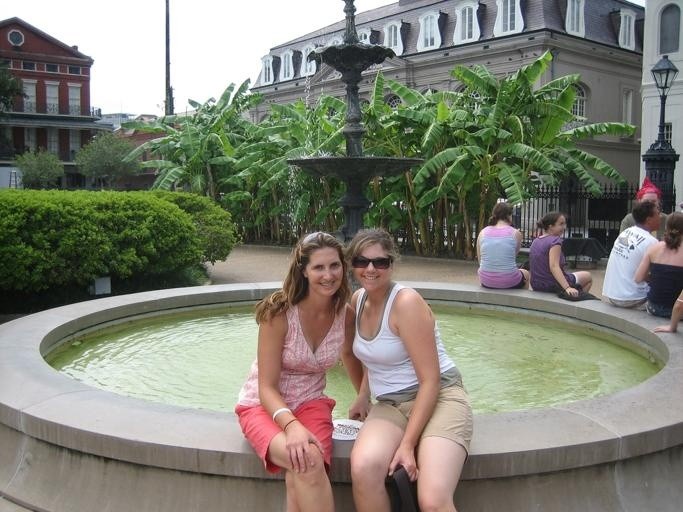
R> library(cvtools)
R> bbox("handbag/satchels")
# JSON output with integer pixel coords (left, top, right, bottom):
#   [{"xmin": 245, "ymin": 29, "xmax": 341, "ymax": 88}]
[{"xmin": 559, "ymin": 284, "xmax": 601, "ymax": 301}]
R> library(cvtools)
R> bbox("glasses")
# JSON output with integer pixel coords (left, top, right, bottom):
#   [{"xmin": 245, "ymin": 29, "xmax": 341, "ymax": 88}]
[{"xmin": 352, "ymin": 255, "xmax": 390, "ymax": 269}]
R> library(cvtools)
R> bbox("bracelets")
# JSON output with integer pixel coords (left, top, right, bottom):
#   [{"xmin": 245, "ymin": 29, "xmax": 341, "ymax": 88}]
[
  {"xmin": 272, "ymin": 408, "xmax": 291, "ymax": 425},
  {"xmin": 283, "ymin": 418, "xmax": 297, "ymax": 431},
  {"xmin": 565, "ymin": 286, "xmax": 570, "ymax": 291}
]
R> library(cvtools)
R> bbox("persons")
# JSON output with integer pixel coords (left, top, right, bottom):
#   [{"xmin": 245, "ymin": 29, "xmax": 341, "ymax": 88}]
[
  {"xmin": 234, "ymin": 230, "xmax": 372, "ymax": 512},
  {"xmin": 620, "ymin": 183, "xmax": 668, "ymax": 242},
  {"xmin": 601, "ymin": 202, "xmax": 661, "ymax": 308},
  {"xmin": 476, "ymin": 202, "xmax": 530, "ymax": 290},
  {"xmin": 528, "ymin": 212, "xmax": 593, "ymax": 297},
  {"xmin": 337, "ymin": 227, "xmax": 474, "ymax": 512},
  {"xmin": 652, "ymin": 289, "xmax": 683, "ymax": 333},
  {"xmin": 635, "ymin": 212, "xmax": 683, "ymax": 318}
]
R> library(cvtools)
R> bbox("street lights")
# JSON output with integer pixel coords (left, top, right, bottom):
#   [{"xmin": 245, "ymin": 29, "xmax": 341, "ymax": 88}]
[{"xmin": 641, "ymin": 55, "xmax": 680, "ymax": 214}]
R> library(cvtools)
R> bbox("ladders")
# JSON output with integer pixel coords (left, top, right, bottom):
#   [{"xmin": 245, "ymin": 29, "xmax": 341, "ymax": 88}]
[{"xmin": 8, "ymin": 171, "xmax": 17, "ymax": 188}]
